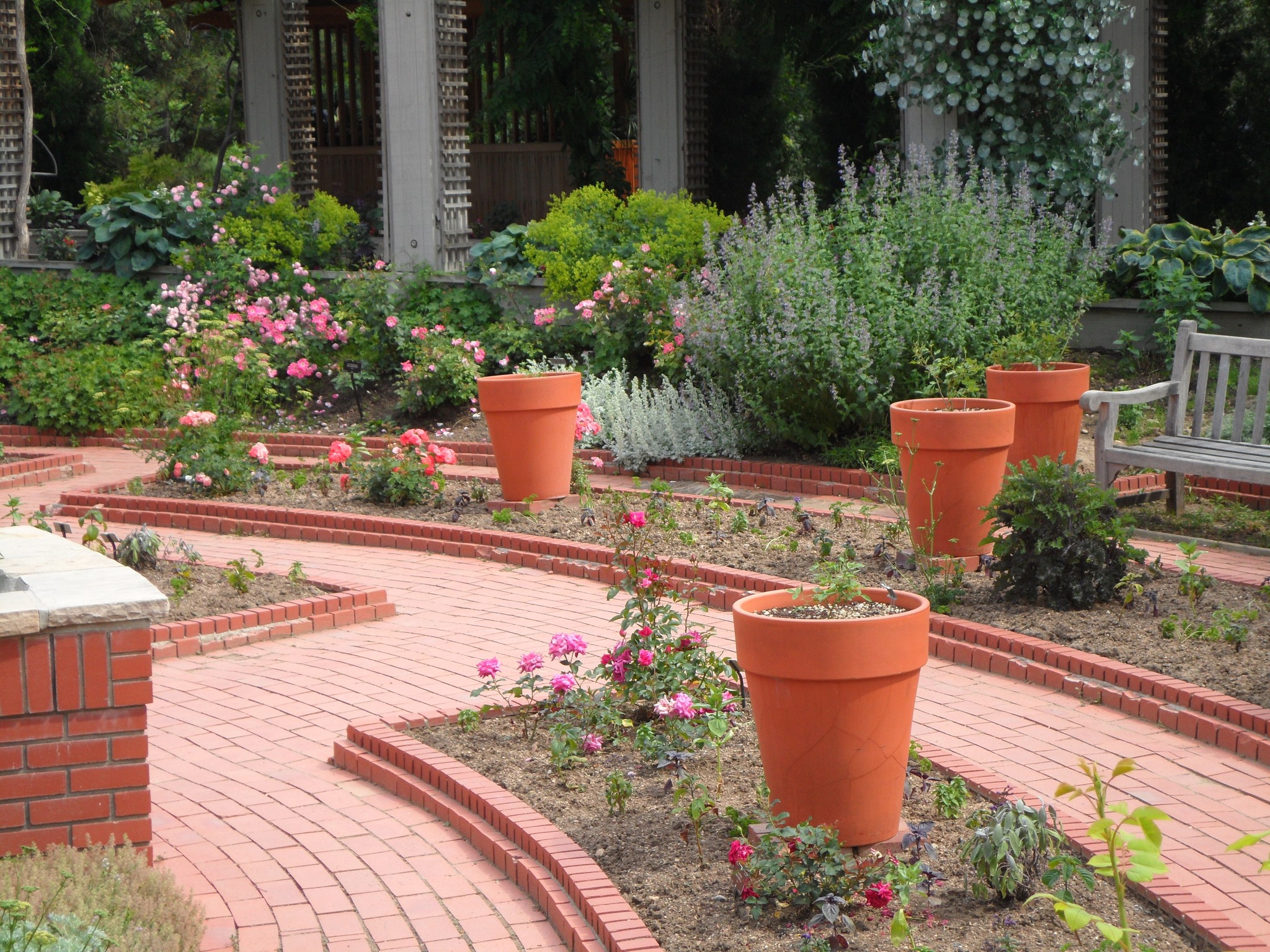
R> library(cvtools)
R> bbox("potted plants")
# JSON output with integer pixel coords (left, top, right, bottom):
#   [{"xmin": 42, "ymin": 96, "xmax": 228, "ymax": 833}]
[
  {"xmin": 889, "ymin": 339, "xmax": 1016, "ymax": 558},
  {"xmin": 984, "ymin": 320, "xmax": 1090, "ymax": 486}
]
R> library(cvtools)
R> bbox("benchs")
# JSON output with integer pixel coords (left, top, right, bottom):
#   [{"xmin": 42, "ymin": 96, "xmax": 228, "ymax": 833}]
[{"xmin": 1079, "ymin": 320, "xmax": 1270, "ymax": 522}]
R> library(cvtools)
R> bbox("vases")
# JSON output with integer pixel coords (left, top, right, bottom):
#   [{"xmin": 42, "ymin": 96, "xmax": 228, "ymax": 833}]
[
  {"xmin": 474, "ymin": 371, "xmax": 582, "ymax": 501},
  {"xmin": 732, "ymin": 584, "xmax": 930, "ymax": 853}
]
